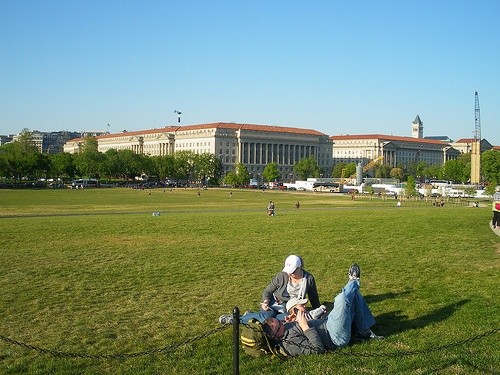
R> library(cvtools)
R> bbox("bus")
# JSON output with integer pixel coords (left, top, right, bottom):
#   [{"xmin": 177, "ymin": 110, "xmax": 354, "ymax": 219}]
[
  {"xmin": 72, "ymin": 179, "xmax": 98, "ymax": 187},
  {"xmin": 313, "ymin": 182, "xmax": 343, "ymax": 193}
]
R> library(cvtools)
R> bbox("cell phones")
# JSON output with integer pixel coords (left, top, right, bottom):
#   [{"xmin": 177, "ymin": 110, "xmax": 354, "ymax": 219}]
[{"xmin": 294, "ymin": 308, "xmax": 298, "ymax": 315}]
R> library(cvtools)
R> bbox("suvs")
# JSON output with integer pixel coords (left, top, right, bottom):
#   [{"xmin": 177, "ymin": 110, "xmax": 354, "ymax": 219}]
[
  {"xmin": 447, "ymin": 190, "xmax": 464, "ymax": 198},
  {"xmin": 50, "ymin": 180, "xmax": 63, "ymax": 187}
]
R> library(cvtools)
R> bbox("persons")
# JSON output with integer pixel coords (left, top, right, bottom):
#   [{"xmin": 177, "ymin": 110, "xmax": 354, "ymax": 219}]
[
  {"xmin": 490, "ymin": 186, "xmax": 500, "ymax": 228},
  {"xmin": 261, "ymin": 262, "xmax": 385, "ymax": 355},
  {"xmin": 285, "ymin": 298, "xmax": 328, "ymax": 322},
  {"xmin": 1, "ymin": 170, "xmax": 490, "ymax": 220},
  {"xmin": 219, "ymin": 254, "xmax": 326, "ymax": 328}
]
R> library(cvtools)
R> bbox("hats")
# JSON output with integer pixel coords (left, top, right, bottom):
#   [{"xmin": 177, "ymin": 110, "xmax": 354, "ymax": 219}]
[
  {"xmin": 282, "ymin": 254, "xmax": 302, "ymax": 274},
  {"xmin": 285, "ymin": 298, "xmax": 308, "ymax": 312}
]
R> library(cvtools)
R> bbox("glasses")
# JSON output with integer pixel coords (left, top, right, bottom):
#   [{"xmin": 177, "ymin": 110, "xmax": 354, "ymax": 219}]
[
  {"xmin": 273, "ymin": 319, "xmax": 281, "ymax": 337},
  {"xmin": 289, "ymin": 267, "xmax": 298, "ymax": 276}
]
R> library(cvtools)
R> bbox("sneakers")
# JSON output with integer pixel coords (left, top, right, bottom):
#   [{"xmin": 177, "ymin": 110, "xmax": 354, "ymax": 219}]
[
  {"xmin": 219, "ymin": 313, "xmax": 243, "ymax": 326},
  {"xmin": 348, "ymin": 263, "xmax": 360, "ymax": 283},
  {"xmin": 354, "ymin": 328, "xmax": 384, "ymax": 342}
]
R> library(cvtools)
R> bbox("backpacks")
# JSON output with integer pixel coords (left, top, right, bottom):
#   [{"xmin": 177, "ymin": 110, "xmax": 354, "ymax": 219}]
[{"xmin": 241, "ymin": 318, "xmax": 271, "ymax": 357}]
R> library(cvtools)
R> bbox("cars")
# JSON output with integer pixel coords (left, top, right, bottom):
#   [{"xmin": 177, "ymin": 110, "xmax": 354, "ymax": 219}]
[
  {"xmin": 381, "ymin": 190, "xmax": 397, "ymax": 196},
  {"xmin": 429, "ymin": 193, "xmax": 442, "ymax": 197},
  {"xmin": 288, "ymin": 187, "xmax": 296, "ymax": 190},
  {"xmin": 234, "ymin": 185, "xmax": 287, "ymax": 191},
  {"xmin": 411, "ymin": 192, "xmax": 425, "ymax": 197},
  {"xmin": 297, "ymin": 187, "xmax": 307, "ymax": 191}
]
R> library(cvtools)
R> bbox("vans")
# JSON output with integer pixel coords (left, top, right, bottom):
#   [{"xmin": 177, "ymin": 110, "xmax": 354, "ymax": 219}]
[{"xmin": 348, "ymin": 189, "xmax": 358, "ymax": 194}]
